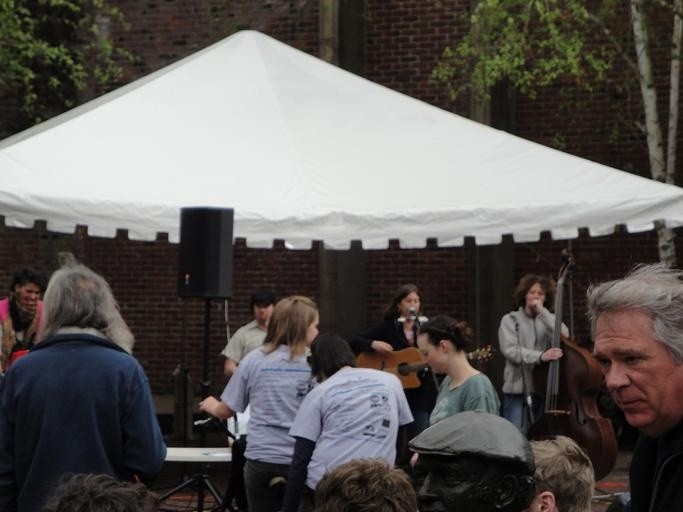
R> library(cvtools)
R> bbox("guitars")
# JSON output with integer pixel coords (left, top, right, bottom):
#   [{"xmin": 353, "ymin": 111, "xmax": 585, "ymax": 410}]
[{"xmin": 356, "ymin": 343, "xmax": 492, "ymax": 386}]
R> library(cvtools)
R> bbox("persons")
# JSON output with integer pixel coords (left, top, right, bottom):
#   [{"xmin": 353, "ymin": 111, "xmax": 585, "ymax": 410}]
[
  {"xmin": 198, "ymin": 295, "xmax": 322, "ymax": 511},
  {"xmin": 218, "ymin": 288, "xmax": 275, "ymax": 377},
  {"xmin": 282, "ymin": 329, "xmax": 429, "ymax": 512},
  {"xmin": 406, "ymin": 407, "xmax": 537, "ymax": 512},
  {"xmin": 417, "ymin": 313, "xmax": 501, "ymax": 428},
  {"xmin": 0, "ymin": 266, "xmax": 47, "ymax": 374},
  {"xmin": 497, "ymin": 272, "xmax": 570, "ymax": 434},
  {"xmin": 43, "ymin": 471, "xmax": 159, "ymax": 511},
  {"xmin": 522, "ymin": 434, "xmax": 596, "ymax": 512},
  {"xmin": 0, "ymin": 251, "xmax": 167, "ymax": 512},
  {"xmin": 585, "ymin": 259, "xmax": 682, "ymax": 512},
  {"xmin": 350, "ymin": 283, "xmax": 442, "ymax": 458},
  {"xmin": 313, "ymin": 456, "xmax": 418, "ymax": 511}
]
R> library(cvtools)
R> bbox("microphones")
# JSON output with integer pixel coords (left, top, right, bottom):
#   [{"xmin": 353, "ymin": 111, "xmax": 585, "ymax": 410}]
[
  {"xmin": 409, "ymin": 306, "xmax": 419, "ymax": 325},
  {"xmin": 508, "ymin": 312, "xmax": 521, "ymax": 331}
]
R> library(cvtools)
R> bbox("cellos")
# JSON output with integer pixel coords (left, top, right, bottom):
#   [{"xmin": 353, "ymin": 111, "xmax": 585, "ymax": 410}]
[{"xmin": 526, "ymin": 249, "xmax": 620, "ymax": 478}]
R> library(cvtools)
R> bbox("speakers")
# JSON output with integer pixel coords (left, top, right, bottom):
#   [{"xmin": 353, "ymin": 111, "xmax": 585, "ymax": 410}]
[{"xmin": 177, "ymin": 207, "xmax": 235, "ymax": 297}]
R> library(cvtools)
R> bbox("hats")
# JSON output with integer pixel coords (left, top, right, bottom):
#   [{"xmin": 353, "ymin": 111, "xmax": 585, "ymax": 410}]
[{"xmin": 411, "ymin": 411, "xmax": 537, "ymax": 469}]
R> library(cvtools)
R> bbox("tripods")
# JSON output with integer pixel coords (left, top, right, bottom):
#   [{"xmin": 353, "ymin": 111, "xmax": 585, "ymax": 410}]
[{"xmin": 157, "ymin": 302, "xmax": 234, "ymax": 511}]
